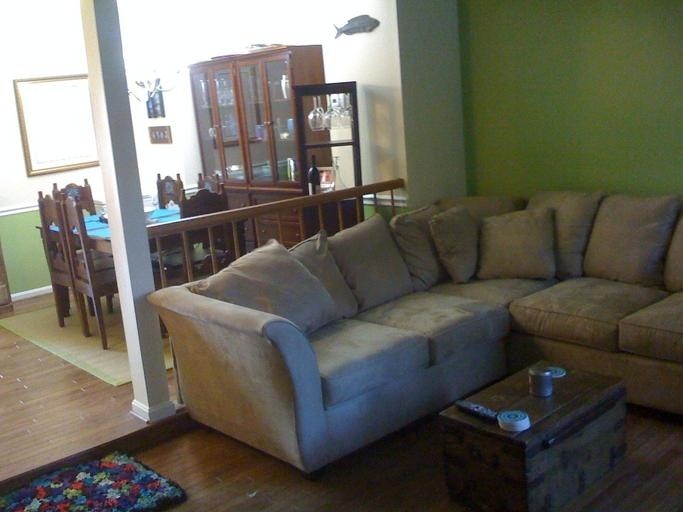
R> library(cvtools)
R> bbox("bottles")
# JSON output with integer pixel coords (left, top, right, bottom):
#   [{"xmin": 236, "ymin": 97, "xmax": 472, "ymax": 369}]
[
  {"xmin": 528, "ymin": 369, "xmax": 555, "ymax": 399},
  {"xmin": 309, "ymin": 152, "xmax": 321, "ymax": 195},
  {"xmin": 281, "ymin": 75, "xmax": 290, "ymax": 100},
  {"xmin": 329, "ymin": 155, "xmax": 350, "ymax": 197}
]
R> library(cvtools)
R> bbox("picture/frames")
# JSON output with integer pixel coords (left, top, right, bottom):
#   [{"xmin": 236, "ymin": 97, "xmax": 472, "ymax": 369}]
[{"xmin": 11, "ymin": 74, "xmax": 100, "ymax": 177}]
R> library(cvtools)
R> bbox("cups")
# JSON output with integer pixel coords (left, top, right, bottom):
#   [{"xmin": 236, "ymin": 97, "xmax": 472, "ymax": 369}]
[{"xmin": 198, "ymin": 79, "xmax": 235, "ymax": 106}]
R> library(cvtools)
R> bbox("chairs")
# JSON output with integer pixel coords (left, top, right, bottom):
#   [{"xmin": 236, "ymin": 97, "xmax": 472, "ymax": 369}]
[{"xmin": 37, "ymin": 172, "xmax": 234, "ymax": 352}]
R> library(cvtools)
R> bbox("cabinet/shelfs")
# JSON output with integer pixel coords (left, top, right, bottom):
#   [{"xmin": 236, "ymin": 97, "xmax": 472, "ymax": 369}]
[{"xmin": 187, "ymin": 44, "xmax": 362, "ymax": 266}]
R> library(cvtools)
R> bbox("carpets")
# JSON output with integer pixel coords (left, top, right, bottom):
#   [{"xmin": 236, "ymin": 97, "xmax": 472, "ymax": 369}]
[
  {"xmin": 1, "ymin": 294, "xmax": 178, "ymax": 388},
  {"xmin": 0, "ymin": 453, "xmax": 187, "ymax": 511}
]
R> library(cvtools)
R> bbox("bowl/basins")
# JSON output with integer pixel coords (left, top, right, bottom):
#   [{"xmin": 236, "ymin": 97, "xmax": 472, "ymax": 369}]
[
  {"xmin": 286, "ymin": 116, "xmax": 294, "ymax": 136},
  {"xmin": 255, "ymin": 124, "xmax": 268, "ymax": 139}
]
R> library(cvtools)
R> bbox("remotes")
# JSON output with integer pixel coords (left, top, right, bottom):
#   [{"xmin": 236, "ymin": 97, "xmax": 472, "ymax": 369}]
[{"xmin": 455, "ymin": 399, "xmax": 498, "ymax": 418}]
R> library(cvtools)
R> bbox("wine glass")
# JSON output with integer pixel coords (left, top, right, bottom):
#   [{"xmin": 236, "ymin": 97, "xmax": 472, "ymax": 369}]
[{"xmin": 309, "ymin": 93, "xmax": 354, "ymax": 131}]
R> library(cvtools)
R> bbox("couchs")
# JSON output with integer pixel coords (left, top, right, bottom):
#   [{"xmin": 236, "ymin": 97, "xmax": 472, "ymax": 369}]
[{"xmin": 150, "ymin": 191, "xmax": 683, "ymax": 484}]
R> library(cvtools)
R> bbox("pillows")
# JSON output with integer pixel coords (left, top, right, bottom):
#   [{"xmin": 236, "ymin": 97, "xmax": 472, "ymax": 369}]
[
  {"xmin": 189, "ymin": 239, "xmax": 339, "ymax": 334},
  {"xmin": 427, "ymin": 207, "xmax": 558, "ymax": 284}
]
